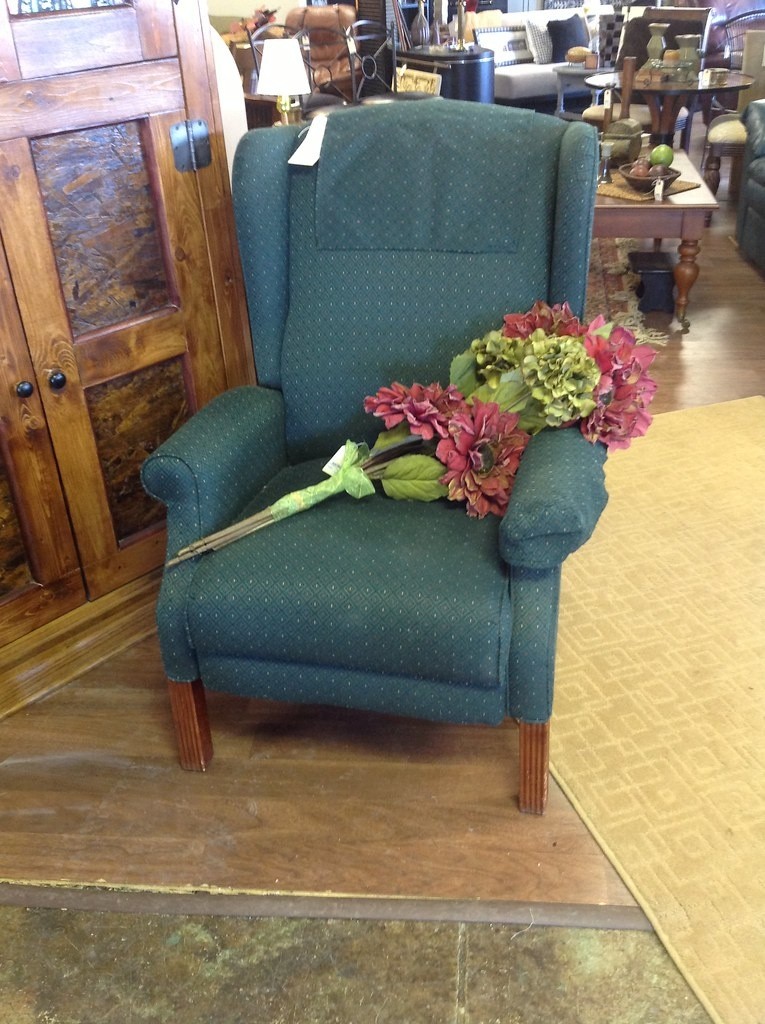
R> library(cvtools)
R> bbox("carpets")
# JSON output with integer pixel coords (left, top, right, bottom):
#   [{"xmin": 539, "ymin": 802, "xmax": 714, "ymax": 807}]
[
  {"xmin": 513, "ymin": 392, "xmax": 765, "ymax": 1024},
  {"xmin": 588, "ymin": 235, "xmax": 670, "ymax": 347}
]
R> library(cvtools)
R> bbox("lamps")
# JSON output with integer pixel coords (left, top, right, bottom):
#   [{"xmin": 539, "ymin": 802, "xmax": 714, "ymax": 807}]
[{"xmin": 253, "ymin": 37, "xmax": 311, "ymax": 126}]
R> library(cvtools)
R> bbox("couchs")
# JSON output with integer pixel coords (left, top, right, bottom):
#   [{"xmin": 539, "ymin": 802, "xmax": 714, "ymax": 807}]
[
  {"xmin": 467, "ymin": 6, "xmax": 618, "ymax": 99},
  {"xmin": 140, "ymin": 98, "xmax": 600, "ymax": 816},
  {"xmin": 733, "ymin": 99, "xmax": 765, "ymax": 279},
  {"xmin": 283, "ymin": 5, "xmax": 363, "ymax": 105}
]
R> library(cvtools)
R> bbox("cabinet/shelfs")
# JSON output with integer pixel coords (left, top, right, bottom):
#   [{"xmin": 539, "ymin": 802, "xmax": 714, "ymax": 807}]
[{"xmin": 0, "ymin": 1, "xmax": 260, "ymax": 649}]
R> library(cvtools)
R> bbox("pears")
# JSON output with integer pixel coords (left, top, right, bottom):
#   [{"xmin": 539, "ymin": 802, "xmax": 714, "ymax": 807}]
[{"xmin": 650, "ymin": 144, "xmax": 673, "ymax": 167}]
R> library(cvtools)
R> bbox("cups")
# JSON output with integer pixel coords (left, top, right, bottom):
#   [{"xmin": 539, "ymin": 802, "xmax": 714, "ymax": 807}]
[{"xmin": 707, "ymin": 67, "xmax": 729, "ymax": 85}]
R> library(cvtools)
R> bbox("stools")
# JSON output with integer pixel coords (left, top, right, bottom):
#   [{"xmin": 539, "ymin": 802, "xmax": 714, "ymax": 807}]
[
  {"xmin": 582, "ymin": 101, "xmax": 691, "ymax": 176},
  {"xmin": 700, "ymin": 113, "xmax": 752, "ymax": 228}
]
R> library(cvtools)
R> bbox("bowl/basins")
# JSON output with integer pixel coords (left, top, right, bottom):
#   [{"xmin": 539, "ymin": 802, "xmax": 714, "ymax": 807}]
[{"xmin": 618, "ymin": 163, "xmax": 681, "ymax": 191}]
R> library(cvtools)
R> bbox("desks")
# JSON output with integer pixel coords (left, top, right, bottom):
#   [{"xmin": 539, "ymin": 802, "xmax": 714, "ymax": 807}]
[
  {"xmin": 585, "ymin": 69, "xmax": 756, "ymax": 138},
  {"xmin": 593, "ymin": 146, "xmax": 719, "ymax": 329},
  {"xmin": 553, "ymin": 66, "xmax": 613, "ymax": 119}
]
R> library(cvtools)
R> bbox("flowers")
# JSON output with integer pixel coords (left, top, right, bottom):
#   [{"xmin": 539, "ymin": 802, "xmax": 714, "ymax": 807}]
[{"xmin": 165, "ymin": 297, "xmax": 670, "ymax": 571}]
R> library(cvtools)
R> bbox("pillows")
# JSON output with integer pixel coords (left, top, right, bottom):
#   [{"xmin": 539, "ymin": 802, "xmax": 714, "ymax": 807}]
[
  {"xmin": 548, "ymin": 14, "xmax": 586, "ymax": 64},
  {"xmin": 476, "ymin": 22, "xmax": 532, "ymax": 67},
  {"xmin": 526, "ymin": 19, "xmax": 553, "ymax": 64}
]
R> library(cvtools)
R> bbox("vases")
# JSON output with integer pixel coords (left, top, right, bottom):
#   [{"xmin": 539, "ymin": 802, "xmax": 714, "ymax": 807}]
[
  {"xmin": 641, "ymin": 24, "xmax": 671, "ymax": 69},
  {"xmin": 675, "ymin": 34, "xmax": 702, "ymax": 81}
]
